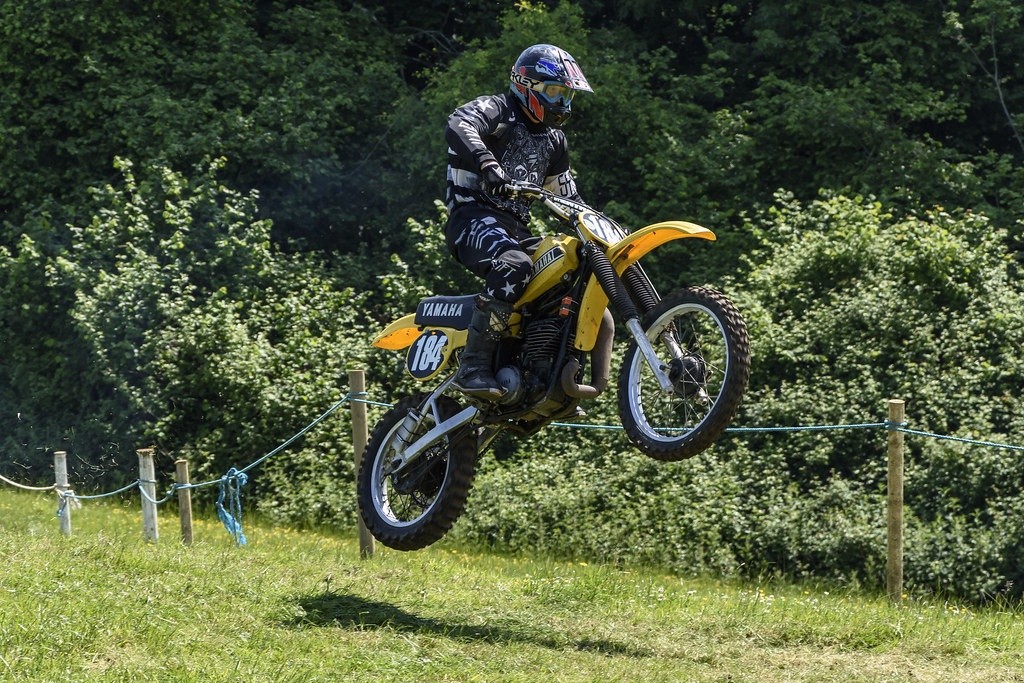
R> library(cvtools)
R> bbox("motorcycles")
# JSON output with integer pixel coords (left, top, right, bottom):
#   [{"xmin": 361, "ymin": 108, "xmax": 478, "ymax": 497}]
[{"xmin": 355, "ymin": 181, "xmax": 754, "ymax": 552}]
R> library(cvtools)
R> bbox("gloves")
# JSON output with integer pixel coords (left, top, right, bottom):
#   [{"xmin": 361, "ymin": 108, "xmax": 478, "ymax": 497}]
[{"xmin": 481, "ymin": 163, "xmax": 512, "ymax": 202}]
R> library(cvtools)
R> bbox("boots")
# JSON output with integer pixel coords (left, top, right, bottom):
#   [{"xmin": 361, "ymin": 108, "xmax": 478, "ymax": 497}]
[{"xmin": 451, "ymin": 293, "xmax": 514, "ymax": 400}]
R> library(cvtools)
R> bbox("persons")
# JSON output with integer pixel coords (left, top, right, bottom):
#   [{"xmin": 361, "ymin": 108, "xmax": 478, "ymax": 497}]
[{"xmin": 445, "ymin": 43, "xmax": 599, "ymax": 390}]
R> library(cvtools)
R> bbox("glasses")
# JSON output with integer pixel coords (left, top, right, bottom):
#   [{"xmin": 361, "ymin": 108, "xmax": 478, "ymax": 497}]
[{"xmin": 511, "ymin": 66, "xmax": 576, "ymax": 106}]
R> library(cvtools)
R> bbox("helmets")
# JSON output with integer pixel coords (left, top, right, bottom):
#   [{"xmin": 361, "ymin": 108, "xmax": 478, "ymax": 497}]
[{"xmin": 510, "ymin": 43, "xmax": 593, "ymax": 129}]
